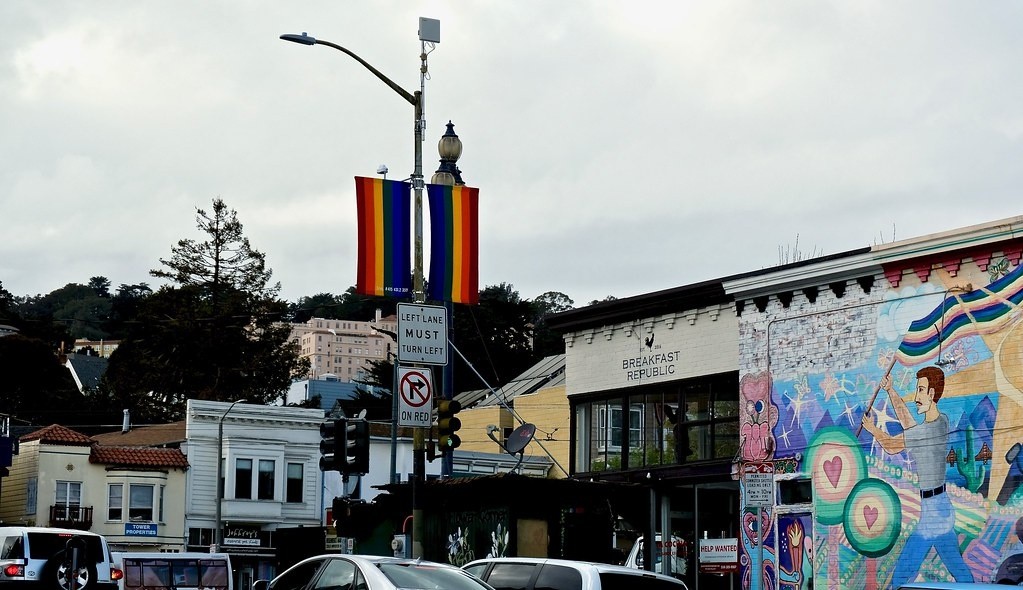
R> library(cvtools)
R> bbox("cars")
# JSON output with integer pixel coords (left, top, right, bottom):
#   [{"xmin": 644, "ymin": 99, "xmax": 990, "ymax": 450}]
[{"xmin": 253, "ymin": 555, "xmax": 496, "ymax": 590}]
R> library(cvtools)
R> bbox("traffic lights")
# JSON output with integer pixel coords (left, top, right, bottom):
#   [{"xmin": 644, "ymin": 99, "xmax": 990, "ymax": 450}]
[
  {"xmin": 318, "ymin": 420, "xmax": 343, "ymax": 472},
  {"xmin": 437, "ymin": 398, "xmax": 461, "ymax": 452}
]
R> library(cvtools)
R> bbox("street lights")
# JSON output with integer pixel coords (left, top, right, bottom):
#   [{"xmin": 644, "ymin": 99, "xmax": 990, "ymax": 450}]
[
  {"xmin": 429, "ymin": 121, "xmax": 464, "ymax": 476},
  {"xmin": 215, "ymin": 400, "xmax": 245, "ymax": 553},
  {"xmin": 280, "ymin": 32, "xmax": 426, "ymax": 558}
]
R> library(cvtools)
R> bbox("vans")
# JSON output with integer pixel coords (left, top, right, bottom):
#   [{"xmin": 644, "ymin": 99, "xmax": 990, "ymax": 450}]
[{"xmin": 459, "ymin": 557, "xmax": 688, "ymax": 590}]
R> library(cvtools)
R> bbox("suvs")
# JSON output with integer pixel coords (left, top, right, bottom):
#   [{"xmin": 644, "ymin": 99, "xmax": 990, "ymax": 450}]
[
  {"xmin": 0, "ymin": 526, "xmax": 119, "ymax": 590},
  {"xmin": 624, "ymin": 534, "xmax": 687, "ymax": 578}
]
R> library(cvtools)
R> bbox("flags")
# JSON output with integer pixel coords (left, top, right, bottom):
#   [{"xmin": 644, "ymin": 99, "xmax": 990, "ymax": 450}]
[
  {"xmin": 426, "ymin": 183, "xmax": 479, "ymax": 304},
  {"xmin": 355, "ymin": 176, "xmax": 413, "ymax": 298}
]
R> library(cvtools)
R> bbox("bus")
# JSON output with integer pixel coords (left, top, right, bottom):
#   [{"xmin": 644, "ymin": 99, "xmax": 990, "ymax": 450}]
[{"xmin": 110, "ymin": 552, "xmax": 233, "ymax": 590}]
